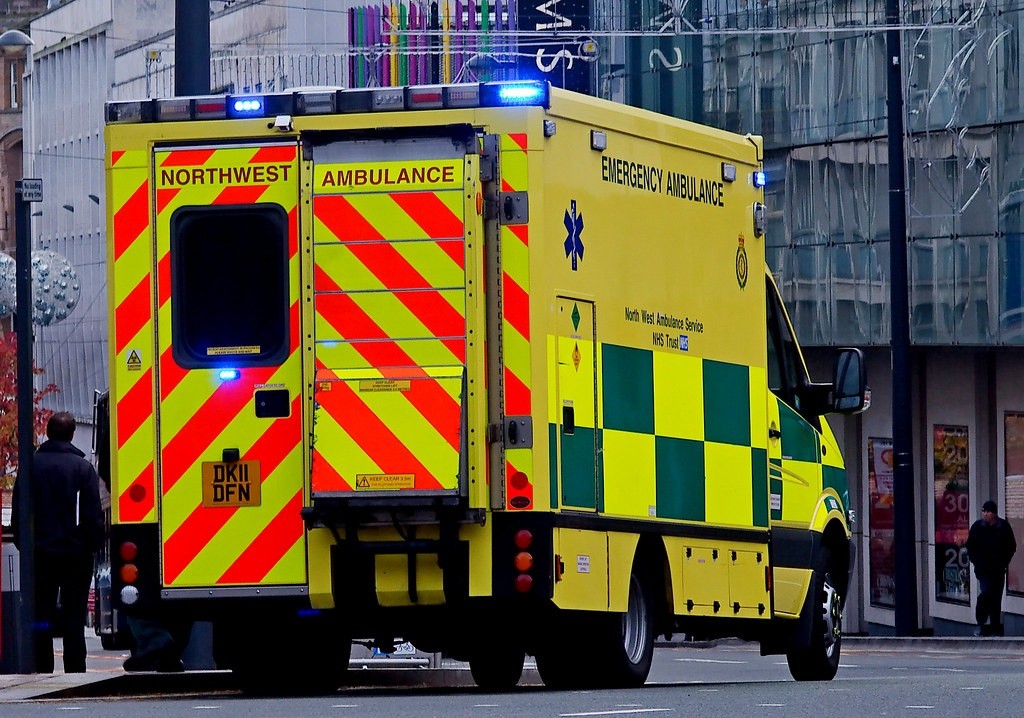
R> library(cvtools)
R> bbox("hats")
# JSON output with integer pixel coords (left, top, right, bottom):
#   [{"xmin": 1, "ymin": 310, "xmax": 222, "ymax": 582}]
[{"xmin": 982, "ymin": 500, "xmax": 997, "ymax": 515}]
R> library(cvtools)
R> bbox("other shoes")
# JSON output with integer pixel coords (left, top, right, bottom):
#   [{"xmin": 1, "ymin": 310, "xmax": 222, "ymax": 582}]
[{"xmin": 974, "ymin": 624, "xmax": 983, "ymax": 636}]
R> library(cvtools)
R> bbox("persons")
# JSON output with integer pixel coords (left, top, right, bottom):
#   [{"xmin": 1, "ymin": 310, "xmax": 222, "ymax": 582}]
[
  {"xmin": 966, "ymin": 500, "xmax": 1017, "ymax": 636},
  {"xmin": 10, "ymin": 411, "xmax": 106, "ymax": 674}
]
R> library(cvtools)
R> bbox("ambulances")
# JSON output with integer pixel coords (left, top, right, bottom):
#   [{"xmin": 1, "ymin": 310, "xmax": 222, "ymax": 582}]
[{"xmin": 98, "ymin": 76, "xmax": 871, "ymax": 696}]
